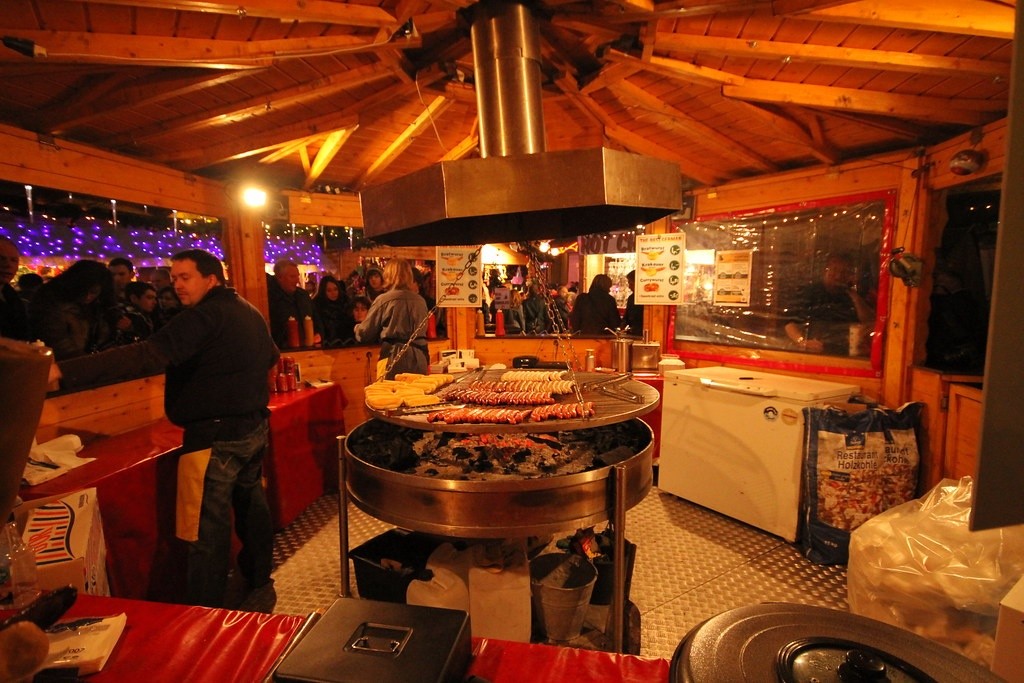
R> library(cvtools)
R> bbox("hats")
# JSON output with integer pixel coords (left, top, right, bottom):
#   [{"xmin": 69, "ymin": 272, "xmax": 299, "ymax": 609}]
[{"xmin": 366, "ymin": 265, "xmax": 383, "ymax": 275}]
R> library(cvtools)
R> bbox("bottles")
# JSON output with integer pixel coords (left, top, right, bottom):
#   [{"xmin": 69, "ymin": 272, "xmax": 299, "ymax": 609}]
[
  {"xmin": 287, "ymin": 316, "xmax": 301, "ymax": 348},
  {"xmin": 476, "ymin": 308, "xmax": 486, "ymax": 336},
  {"xmin": 425, "ymin": 310, "xmax": 437, "ymax": 339},
  {"xmin": 0, "ymin": 521, "xmax": 42, "ymax": 620},
  {"xmin": 495, "ymin": 307, "xmax": 506, "ymax": 336},
  {"xmin": 404, "ymin": 540, "xmax": 479, "ymax": 614},
  {"xmin": 585, "ymin": 349, "xmax": 595, "ymax": 372},
  {"xmin": 303, "ymin": 315, "xmax": 314, "ymax": 346}
]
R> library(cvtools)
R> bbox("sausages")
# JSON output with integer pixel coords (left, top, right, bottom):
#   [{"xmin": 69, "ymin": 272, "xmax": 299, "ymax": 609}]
[{"xmin": 428, "ymin": 370, "xmax": 594, "ymax": 422}]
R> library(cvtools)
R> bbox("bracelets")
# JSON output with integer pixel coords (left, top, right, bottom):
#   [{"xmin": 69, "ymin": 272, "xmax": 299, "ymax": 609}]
[{"xmin": 796, "ymin": 336, "xmax": 804, "ymax": 344}]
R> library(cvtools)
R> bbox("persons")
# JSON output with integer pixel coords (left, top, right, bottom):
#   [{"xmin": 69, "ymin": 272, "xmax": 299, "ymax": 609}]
[
  {"xmin": 780, "ymin": 251, "xmax": 873, "ymax": 355},
  {"xmin": 569, "ymin": 273, "xmax": 619, "ymax": 336},
  {"xmin": 354, "ymin": 258, "xmax": 433, "ymax": 378},
  {"xmin": 43, "ymin": 249, "xmax": 280, "ymax": 608},
  {"xmin": 624, "ymin": 270, "xmax": 643, "ymax": 336},
  {"xmin": 0, "ymin": 236, "xmax": 579, "ymax": 373}
]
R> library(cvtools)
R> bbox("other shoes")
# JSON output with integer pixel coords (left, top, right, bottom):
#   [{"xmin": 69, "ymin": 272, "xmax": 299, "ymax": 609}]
[
  {"xmin": 151, "ymin": 575, "xmax": 182, "ymax": 604},
  {"xmin": 239, "ymin": 579, "xmax": 276, "ymax": 611}
]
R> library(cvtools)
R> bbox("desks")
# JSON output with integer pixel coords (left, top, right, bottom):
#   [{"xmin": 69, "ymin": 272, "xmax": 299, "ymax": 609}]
[
  {"xmin": 0, "ymin": 596, "xmax": 671, "ymax": 683},
  {"xmin": 20, "ymin": 381, "xmax": 348, "ymax": 600}
]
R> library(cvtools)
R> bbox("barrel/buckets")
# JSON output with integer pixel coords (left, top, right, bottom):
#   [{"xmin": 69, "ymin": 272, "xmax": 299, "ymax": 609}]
[{"xmin": 529, "ymin": 552, "xmax": 599, "ymax": 641}]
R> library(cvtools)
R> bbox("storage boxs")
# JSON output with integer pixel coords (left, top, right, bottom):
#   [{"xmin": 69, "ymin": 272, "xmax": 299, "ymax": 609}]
[{"xmin": 22, "ymin": 487, "xmax": 112, "ymax": 596}]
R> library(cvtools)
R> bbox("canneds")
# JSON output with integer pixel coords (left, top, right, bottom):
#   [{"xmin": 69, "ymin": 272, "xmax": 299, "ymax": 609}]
[
  {"xmin": 278, "ymin": 358, "xmax": 284, "ymax": 372},
  {"xmin": 278, "ymin": 374, "xmax": 288, "ymax": 391},
  {"xmin": 287, "ymin": 374, "xmax": 297, "ymax": 390},
  {"xmin": 270, "ymin": 375, "xmax": 277, "ymax": 391},
  {"xmin": 284, "ymin": 357, "xmax": 295, "ymax": 373}
]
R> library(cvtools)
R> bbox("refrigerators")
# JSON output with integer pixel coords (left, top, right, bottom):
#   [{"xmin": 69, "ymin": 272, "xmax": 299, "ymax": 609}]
[{"xmin": 658, "ymin": 365, "xmax": 861, "ymax": 544}]
